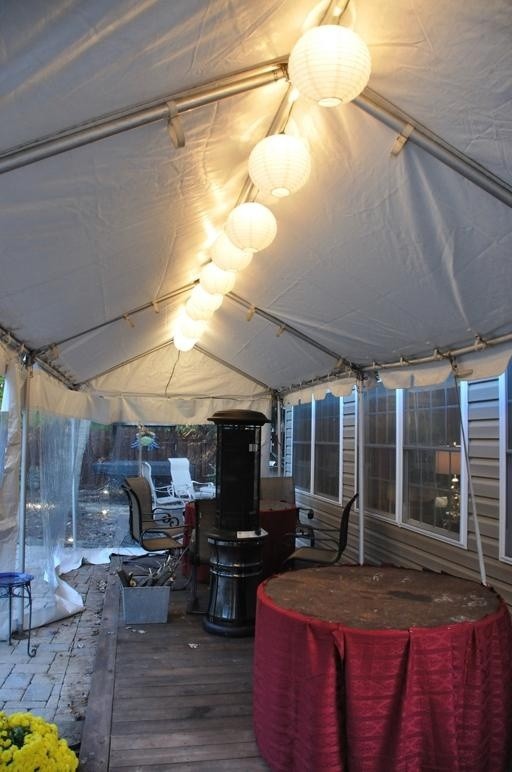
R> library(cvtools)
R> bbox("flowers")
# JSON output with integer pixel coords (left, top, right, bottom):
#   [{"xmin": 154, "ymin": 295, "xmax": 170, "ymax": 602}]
[{"xmin": 0, "ymin": 711, "xmax": 79, "ymax": 772}]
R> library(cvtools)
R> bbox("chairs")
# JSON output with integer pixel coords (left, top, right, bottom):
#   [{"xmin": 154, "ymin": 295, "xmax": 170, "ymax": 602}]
[
  {"xmin": 258, "ymin": 477, "xmax": 315, "ymax": 546},
  {"xmin": 120, "ymin": 457, "xmax": 216, "ymax": 564},
  {"xmin": 281, "ymin": 493, "xmax": 359, "ymax": 566}
]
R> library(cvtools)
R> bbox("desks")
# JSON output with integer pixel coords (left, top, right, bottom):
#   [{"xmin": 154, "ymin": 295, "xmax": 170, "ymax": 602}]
[
  {"xmin": 252, "ymin": 567, "xmax": 512, "ymax": 772},
  {"xmin": 0, "ymin": 572, "xmax": 40, "ymax": 657},
  {"xmin": 183, "ymin": 499, "xmax": 297, "ymax": 584}
]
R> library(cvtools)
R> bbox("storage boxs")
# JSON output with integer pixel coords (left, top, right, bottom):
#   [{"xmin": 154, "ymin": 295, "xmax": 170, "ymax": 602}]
[{"xmin": 122, "ymin": 576, "xmax": 171, "ymax": 625}]
